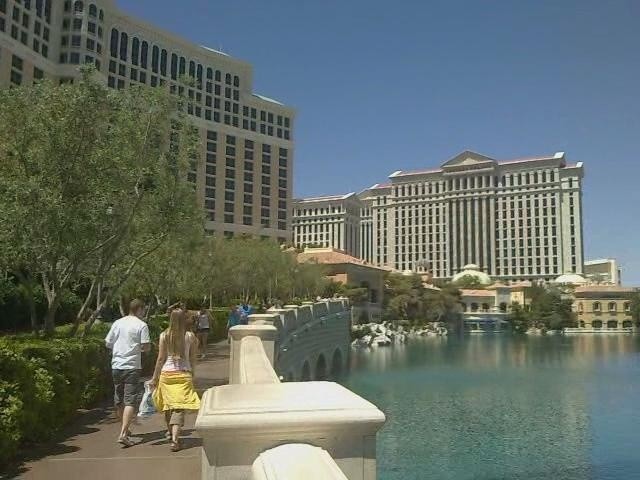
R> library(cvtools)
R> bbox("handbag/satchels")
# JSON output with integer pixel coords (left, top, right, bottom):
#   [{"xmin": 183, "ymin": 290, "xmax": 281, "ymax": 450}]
[{"xmin": 137, "ymin": 380, "xmax": 157, "ymax": 418}]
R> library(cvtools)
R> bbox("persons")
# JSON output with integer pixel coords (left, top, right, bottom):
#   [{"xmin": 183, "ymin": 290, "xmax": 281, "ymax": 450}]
[
  {"xmin": 150, "ymin": 308, "xmax": 197, "ymax": 452},
  {"xmin": 105, "ymin": 298, "xmax": 151, "ymax": 446},
  {"xmin": 166, "ymin": 296, "xmax": 285, "ymax": 359}
]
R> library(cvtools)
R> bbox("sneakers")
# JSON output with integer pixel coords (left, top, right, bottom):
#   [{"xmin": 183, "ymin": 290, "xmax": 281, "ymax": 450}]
[{"xmin": 117, "ymin": 430, "xmax": 182, "ymax": 450}]
